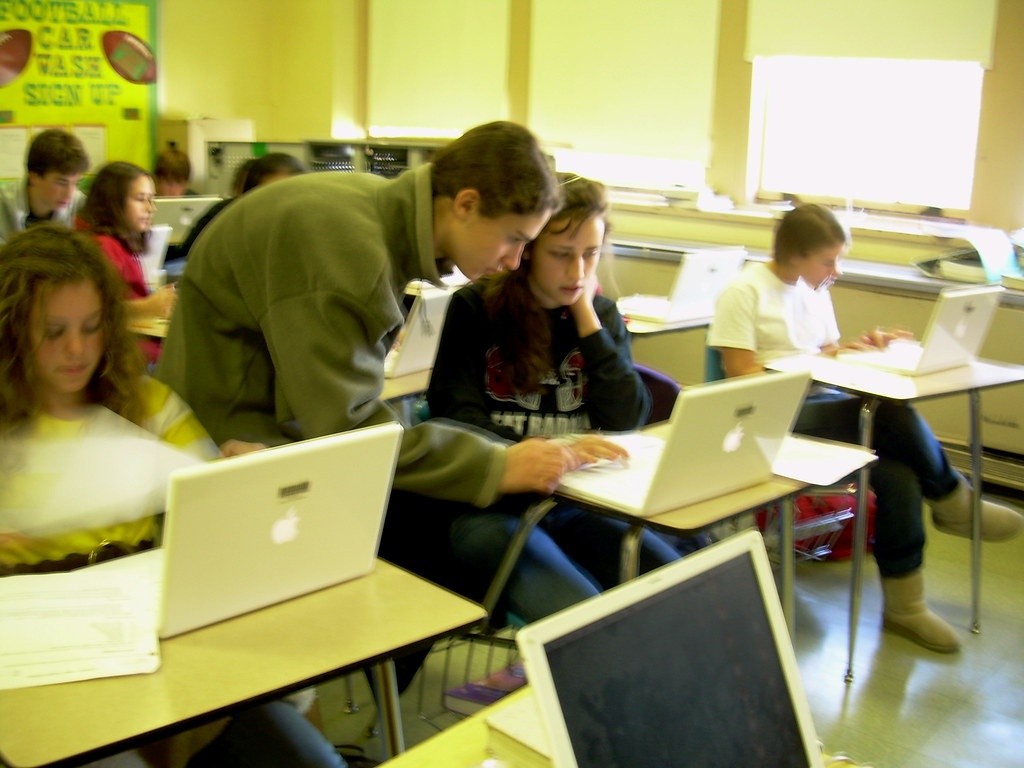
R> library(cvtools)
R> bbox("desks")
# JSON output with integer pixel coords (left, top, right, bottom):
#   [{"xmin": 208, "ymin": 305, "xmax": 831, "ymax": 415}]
[
  {"xmin": 764, "ymin": 342, "xmax": 1024, "ymax": 682},
  {"xmin": 375, "ymin": 683, "xmax": 554, "ymax": 768},
  {"xmin": 555, "ymin": 419, "xmax": 876, "ymax": 652},
  {"xmin": 616, "ymin": 302, "xmax": 710, "ymax": 339},
  {"xmin": 0, "ymin": 546, "xmax": 488, "ymax": 768}
]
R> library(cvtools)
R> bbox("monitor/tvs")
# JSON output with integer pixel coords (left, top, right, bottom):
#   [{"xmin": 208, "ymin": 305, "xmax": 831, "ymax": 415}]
[{"xmin": 514, "ymin": 531, "xmax": 829, "ymax": 768}]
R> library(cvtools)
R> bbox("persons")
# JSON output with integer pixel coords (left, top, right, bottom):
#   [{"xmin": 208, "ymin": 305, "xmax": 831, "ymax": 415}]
[
  {"xmin": 706, "ymin": 203, "xmax": 1024, "ymax": 654},
  {"xmin": 0, "ymin": 120, "xmax": 577, "ymax": 768},
  {"xmin": 424, "ymin": 173, "xmax": 684, "ymax": 631}
]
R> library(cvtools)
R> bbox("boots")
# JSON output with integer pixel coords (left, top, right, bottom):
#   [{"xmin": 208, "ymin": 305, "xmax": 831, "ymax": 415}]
[
  {"xmin": 921, "ymin": 471, "xmax": 1023, "ymax": 543},
  {"xmin": 879, "ymin": 566, "xmax": 963, "ymax": 653}
]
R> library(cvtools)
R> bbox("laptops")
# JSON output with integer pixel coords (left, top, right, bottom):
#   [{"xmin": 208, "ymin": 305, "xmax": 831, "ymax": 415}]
[
  {"xmin": 556, "ymin": 369, "xmax": 813, "ymax": 517},
  {"xmin": 148, "ymin": 198, "xmax": 226, "ymax": 245},
  {"xmin": 383, "ymin": 286, "xmax": 463, "ymax": 379},
  {"xmin": 617, "ymin": 248, "xmax": 748, "ymax": 316},
  {"xmin": 70, "ymin": 420, "xmax": 403, "ymax": 639},
  {"xmin": 838, "ymin": 287, "xmax": 1005, "ymax": 377}
]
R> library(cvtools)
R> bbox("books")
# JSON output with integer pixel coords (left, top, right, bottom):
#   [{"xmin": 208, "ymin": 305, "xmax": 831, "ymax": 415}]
[
  {"xmin": 912, "ymin": 227, "xmax": 1024, "ymax": 290},
  {"xmin": 443, "ymin": 659, "xmax": 528, "ymax": 716},
  {"xmin": 486, "ymin": 696, "xmax": 552, "ymax": 768}
]
